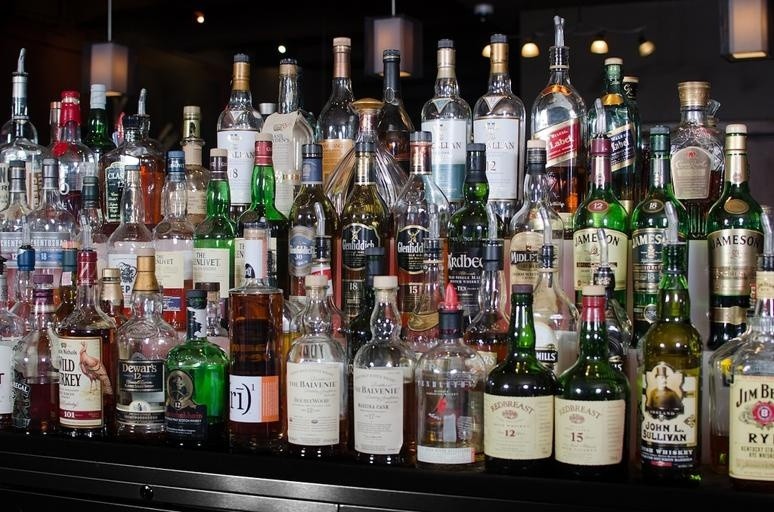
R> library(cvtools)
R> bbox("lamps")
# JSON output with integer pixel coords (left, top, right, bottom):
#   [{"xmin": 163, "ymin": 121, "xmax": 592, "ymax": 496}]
[
  {"xmin": 720, "ymin": 0, "xmax": 774, "ymax": 64},
  {"xmin": 90, "ymin": 0, "xmax": 129, "ymax": 97},
  {"xmin": 371, "ymin": 0, "xmax": 413, "ymax": 78}
]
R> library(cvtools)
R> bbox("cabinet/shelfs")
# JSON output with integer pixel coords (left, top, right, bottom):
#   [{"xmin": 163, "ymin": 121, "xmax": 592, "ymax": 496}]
[{"xmin": 1, "ymin": 434, "xmax": 774, "ymax": 511}]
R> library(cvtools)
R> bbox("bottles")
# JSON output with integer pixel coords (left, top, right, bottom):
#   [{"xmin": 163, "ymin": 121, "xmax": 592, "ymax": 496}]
[
  {"xmin": 732, "ymin": 253, "xmax": 773, "ymax": 492},
  {"xmin": 99, "ymin": 267, "xmax": 128, "ymax": 328},
  {"xmin": 0, "ymin": 48, "xmax": 39, "ymax": 143},
  {"xmin": 631, "ymin": 124, "xmax": 689, "ymax": 347},
  {"xmin": 165, "ymin": 291, "xmax": 229, "ymax": 447},
  {"xmin": 578, "ymin": 228, "xmax": 634, "ymax": 366},
  {"xmin": 707, "ymin": 283, "xmax": 756, "ymax": 476},
  {"xmin": 133, "ymin": 89, "xmax": 168, "ymax": 166},
  {"xmin": 760, "ymin": 205, "xmax": 774, "ymax": 271},
  {"xmin": 375, "ymin": 47, "xmax": 417, "ymax": 182},
  {"xmin": 473, "ymin": 35, "xmax": 525, "ymax": 238},
  {"xmin": 11, "ymin": 277, "xmax": 59, "ymax": 436},
  {"xmin": 391, "ymin": 131, "xmax": 455, "ymax": 328},
  {"xmin": 58, "ymin": 248, "xmax": 79, "ymax": 327},
  {"xmin": 193, "ymin": 148, "xmax": 235, "ymax": 329},
  {"xmin": 150, "ymin": 152, "xmax": 196, "ymax": 342},
  {"xmin": 707, "ymin": 100, "xmax": 725, "ymax": 148},
  {"xmin": 56, "ymin": 250, "xmax": 117, "ymax": 443},
  {"xmin": 587, "ymin": 58, "xmax": 638, "ymax": 200},
  {"xmin": 531, "ymin": 17, "xmax": 587, "ymax": 212},
  {"xmin": 26, "ymin": 159, "xmax": 79, "ymax": 307},
  {"xmin": 106, "ymin": 164, "xmax": 154, "ymax": 321},
  {"xmin": 216, "ymin": 55, "xmax": 264, "ymax": 221},
  {"xmin": 449, "ymin": 144, "xmax": 505, "ymax": 323},
  {"xmin": 482, "ymin": 283, "xmax": 555, "ymax": 476},
  {"xmin": 420, "ymin": 39, "xmax": 473, "ymax": 213},
  {"xmin": 415, "ymin": 282, "xmax": 487, "ymax": 469},
  {"xmin": 45, "ymin": 91, "xmax": 97, "ymax": 222},
  {"xmin": 240, "ymin": 133, "xmax": 291, "ymax": 302},
  {"xmin": 465, "ymin": 240, "xmax": 511, "ymax": 374},
  {"xmin": 98, "ymin": 115, "xmax": 167, "ymax": 237},
  {"xmin": 322, "ymin": 98, "xmax": 409, "ymax": 217},
  {"xmin": 46, "ymin": 101, "xmax": 63, "ymax": 154},
  {"xmin": 259, "ymin": 101, "xmax": 278, "ymax": 126},
  {"xmin": 260, "ymin": 59, "xmax": 315, "ymax": 219},
  {"xmin": 337, "ymin": 143, "xmax": 391, "ymax": 326},
  {"xmin": 288, "ymin": 142, "xmax": 340, "ymax": 311},
  {"xmin": 638, "ymin": 245, "xmax": 702, "ymax": 482},
  {"xmin": 556, "ymin": 286, "xmax": 629, "ymax": 482},
  {"xmin": 708, "ymin": 124, "xmax": 764, "ymax": 347},
  {"xmin": 229, "ymin": 223, "xmax": 286, "ymax": 456},
  {"xmin": 530, "ymin": 207, "xmax": 581, "ymax": 375},
  {"xmin": 161, "ymin": 107, "xmax": 209, "ymax": 232},
  {"xmin": 78, "ymin": 176, "xmax": 109, "ymax": 280},
  {"xmin": 509, "ymin": 138, "xmax": 564, "ymax": 293},
  {"xmin": 622, "ymin": 76, "xmax": 638, "ymax": 115},
  {"xmin": 113, "ymin": 248, "xmax": 177, "ymax": 440},
  {"xmin": 6, "ymin": 244, "xmax": 36, "ymax": 321},
  {"xmin": 1, "ymin": 161, "xmax": 33, "ymax": 275},
  {"xmin": 316, "ymin": 38, "xmax": 360, "ymax": 191},
  {"xmin": 572, "ymin": 132, "xmax": 630, "ymax": 316},
  {"xmin": 407, "ymin": 238, "xmax": 447, "ymax": 353},
  {"xmin": 670, "ymin": 82, "xmax": 725, "ymax": 240},
  {"xmin": 353, "ymin": 276, "xmax": 416, "ymax": 463},
  {"xmin": 0, "ymin": 254, "xmax": 25, "ymax": 429},
  {"xmin": 0, "ymin": 115, "xmax": 46, "ymax": 211},
  {"xmin": 285, "ymin": 275, "xmax": 348, "ymax": 459}
]
